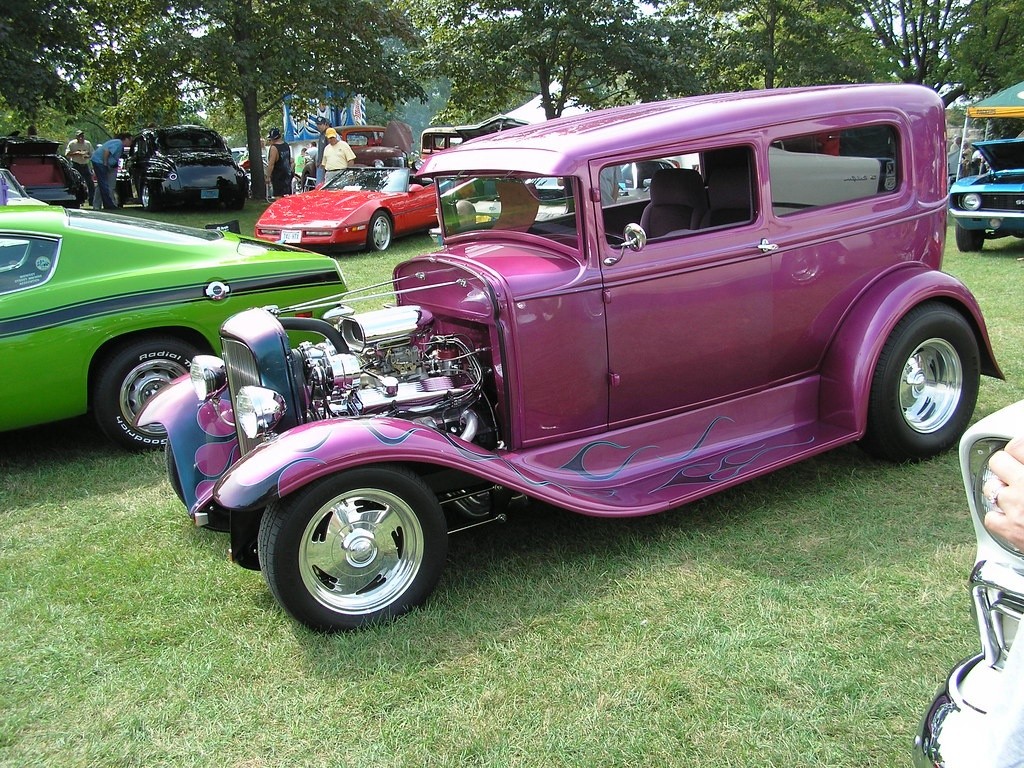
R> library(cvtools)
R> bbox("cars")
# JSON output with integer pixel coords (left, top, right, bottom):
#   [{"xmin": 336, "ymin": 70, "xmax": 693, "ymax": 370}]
[
  {"xmin": 301, "ymin": 125, "xmax": 423, "ymax": 192},
  {"xmin": 0, "ymin": 135, "xmax": 89, "ymax": 205},
  {"xmin": 94, "ymin": 126, "xmax": 249, "ymax": 212},
  {"xmin": 949, "ymin": 138, "xmax": 1024, "ymax": 252},
  {"xmin": 136, "ymin": 83, "xmax": 1007, "ymax": 634},
  {"xmin": 420, "ymin": 126, "xmax": 701, "ymax": 245}
]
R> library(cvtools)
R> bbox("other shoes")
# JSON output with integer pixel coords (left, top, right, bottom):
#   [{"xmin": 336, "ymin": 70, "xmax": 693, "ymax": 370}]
[
  {"xmin": 105, "ymin": 205, "xmax": 122, "ymax": 210},
  {"xmin": 89, "ymin": 203, "xmax": 94, "ymax": 207}
]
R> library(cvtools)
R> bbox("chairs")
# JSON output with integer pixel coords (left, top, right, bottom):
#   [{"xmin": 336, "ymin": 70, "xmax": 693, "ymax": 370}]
[
  {"xmin": 701, "ymin": 168, "xmax": 755, "ymax": 231},
  {"xmin": 641, "ymin": 167, "xmax": 706, "ymax": 236},
  {"xmin": 410, "ymin": 174, "xmax": 423, "ymax": 184}
]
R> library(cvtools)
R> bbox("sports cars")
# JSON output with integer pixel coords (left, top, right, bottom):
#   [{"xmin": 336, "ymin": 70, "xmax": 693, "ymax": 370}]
[
  {"xmin": 254, "ymin": 166, "xmax": 478, "ymax": 254},
  {"xmin": 0, "ymin": 205, "xmax": 349, "ymax": 452}
]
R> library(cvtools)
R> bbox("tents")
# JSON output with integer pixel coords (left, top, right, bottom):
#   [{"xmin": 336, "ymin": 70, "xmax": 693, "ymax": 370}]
[
  {"xmin": 956, "ymin": 81, "xmax": 1024, "ymax": 182},
  {"xmin": 505, "ymin": 74, "xmax": 600, "ymax": 190}
]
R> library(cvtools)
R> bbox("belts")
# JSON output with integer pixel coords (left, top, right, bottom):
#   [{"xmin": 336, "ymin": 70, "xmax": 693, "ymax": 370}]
[{"xmin": 325, "ymin": 169, "xmax": 340, "ymax": 171}]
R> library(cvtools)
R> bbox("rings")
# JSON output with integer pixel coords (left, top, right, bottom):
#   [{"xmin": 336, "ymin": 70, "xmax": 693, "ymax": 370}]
[{"xmin": 987, "ymin": 484, "xmax": 1006, "ymax": 504}]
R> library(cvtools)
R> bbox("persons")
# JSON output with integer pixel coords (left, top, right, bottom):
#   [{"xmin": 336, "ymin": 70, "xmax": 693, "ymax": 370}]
[
  {"xmin": 64, "ymin": 130, "xmax": 96, "ymax": 206},
  {"xmin": 322, "ymin": 128, "xmax": 357, "ymax": 183},
  {"xmin": 557, "ymin": 166, "xmax": 620, "ymax": 207},
  {"xmin": 968, "ymin": 438, "xmax": 1024, "ymax": 768},
  {"xmin": 236, "ymin": 138, "xmax": 319, "ymax": 199},
  {"xmin": 90, "ymin": 132, "xmax": 134, "ymax": 210},
  {"xmin": 314, "ymin": 116, "xmax": 342, "ymax": 186},
  {"xmin": 264, "ymin": 127, "xmax": 292, "ymax": 201},
  {"xmin": 950, "ymin": 134, "xmax": 987, "ymax": 178}
]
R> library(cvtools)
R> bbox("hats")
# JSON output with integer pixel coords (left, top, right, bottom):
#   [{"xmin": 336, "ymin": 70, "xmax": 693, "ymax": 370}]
[
  {"xmin": 75, "ymin": 129, "xmax": 84, "ymax": 136},
  {"xmin": 315, "ymin": 117, "xmax": 326, "ymax": 124},
  {"xmin": 268, "ymin": 128, "xmax": 280, "ymax": 139},
  {"xmin": 325, "ymin": 127, "xmax": 337, "ymax": 138}
]
